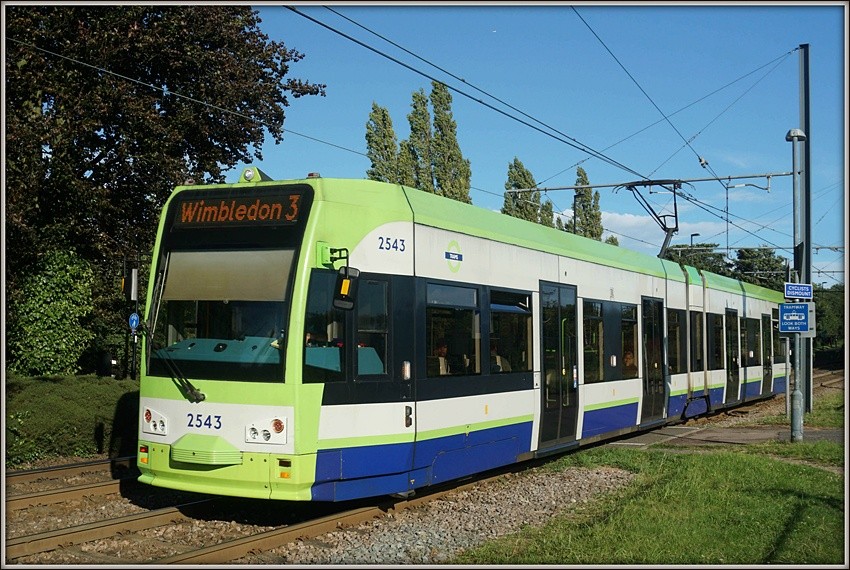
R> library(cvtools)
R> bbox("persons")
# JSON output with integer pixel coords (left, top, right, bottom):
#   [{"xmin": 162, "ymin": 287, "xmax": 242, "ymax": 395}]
[
  {"xmin": 305, "ymin": 325, "xmax": 513, "ymax": 375},
  {"xmin": 622, "ymin": 352, "xmax": 638, "ymax": 379}
]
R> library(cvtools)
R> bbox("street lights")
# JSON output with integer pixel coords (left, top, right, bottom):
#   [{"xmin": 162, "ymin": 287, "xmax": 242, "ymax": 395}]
[
  {"xmin": 690, "ymin": 233, "xmax": 700, "ymax": 266},
  {"xmin": 785, "ymin": 128, "xmax": 806, "ymax": 443}
]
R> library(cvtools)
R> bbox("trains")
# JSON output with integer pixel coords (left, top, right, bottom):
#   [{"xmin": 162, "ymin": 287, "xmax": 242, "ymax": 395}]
[{"xmin": 122, "ymin": 167, "xmax": 791, "ymax": 502}]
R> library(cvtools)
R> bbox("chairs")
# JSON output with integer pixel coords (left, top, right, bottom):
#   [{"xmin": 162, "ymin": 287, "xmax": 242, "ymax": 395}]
[{"xmin": 427, "ymin": 355, "xmax": 503, "ymax": 376}]
